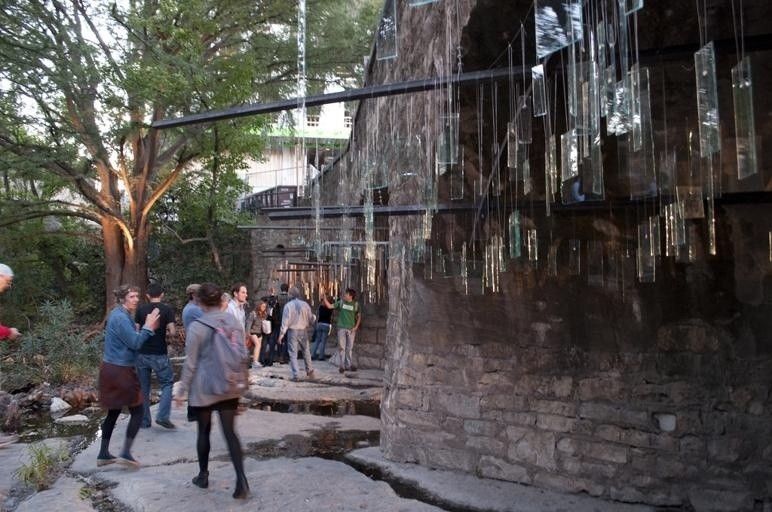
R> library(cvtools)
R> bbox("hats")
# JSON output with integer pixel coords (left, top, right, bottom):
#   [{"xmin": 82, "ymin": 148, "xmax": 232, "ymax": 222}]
[{"xmin": 186, "ymin": 284, "xmax": 202, "ymax": 294}]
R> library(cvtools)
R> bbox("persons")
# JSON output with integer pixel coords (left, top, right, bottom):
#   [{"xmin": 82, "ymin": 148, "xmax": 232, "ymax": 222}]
[
  {"xmin": 0, "ymin": 263, "xmax": 24, "ymax": 344},
  {"xmin": 92, "ymin": 281, "xmax": 162, "ymax": 471},
  {"xmin": 182, "ymin": 282, "xmax": 363, "ymax": 383},
  {"xmin": 177, "ymin": 280, "xmax": 265, "ymax": 501},
  {"xmin": 133, "ymin": 281, "xmax": 178, "ymax": 431}
]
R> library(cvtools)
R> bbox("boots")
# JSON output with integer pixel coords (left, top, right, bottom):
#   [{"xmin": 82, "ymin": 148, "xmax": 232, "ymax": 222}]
[
  {"xmin": 192, "ymin": 471, "xmax": 209, "ymax": 488},
  {"xmin": 97, "ymin": 438, "xmax": 118, "ymax": 467},
  {"xmin": 117, "ymin": 436, "xmax": 140, "ymax": 466},
  {"xmin": 233, "ymin": 476, "xmax": 249, "ymax": 498}
]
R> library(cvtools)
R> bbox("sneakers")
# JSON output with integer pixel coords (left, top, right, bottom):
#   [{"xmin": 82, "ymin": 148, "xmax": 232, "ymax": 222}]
[{"xmin": 155, "ymin": 418, "xmax": 173, "ymax": 428}]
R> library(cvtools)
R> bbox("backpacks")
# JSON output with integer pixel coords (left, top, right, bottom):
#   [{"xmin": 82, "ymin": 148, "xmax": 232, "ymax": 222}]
[{"xmin": 193, "ymin": 319, "xmax": 249, "ymax": 395}]
